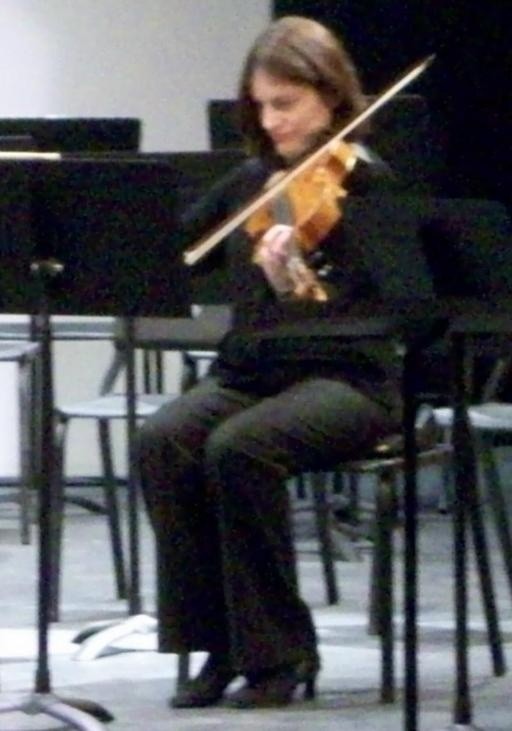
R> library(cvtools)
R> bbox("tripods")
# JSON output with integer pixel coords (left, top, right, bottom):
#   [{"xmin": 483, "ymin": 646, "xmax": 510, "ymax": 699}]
[
  {"xmin": 0, "ymin": 261, "xmax": 112, "ymax": 731},
  {"xmin": 1, "ymin": 311, "xmax": 108, "ymax": 545}
]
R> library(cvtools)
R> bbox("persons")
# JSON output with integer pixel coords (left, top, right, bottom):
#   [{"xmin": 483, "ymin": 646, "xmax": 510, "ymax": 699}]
[{"xmin": 135, "ymin": 12, "xmax": 437, "ymax": 709}]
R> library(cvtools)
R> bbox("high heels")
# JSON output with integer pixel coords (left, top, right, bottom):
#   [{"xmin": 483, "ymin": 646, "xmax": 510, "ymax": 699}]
[
  {"xmin": 173, "ymin": 651, "xmax": 243, "ymax": 708},
  {"xmin": 224, "ymin": 655, "xmax": 319, "ymax": 708}
]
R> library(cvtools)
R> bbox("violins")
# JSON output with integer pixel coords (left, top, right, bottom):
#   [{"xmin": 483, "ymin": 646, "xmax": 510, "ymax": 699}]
[{"xmin": 242, "ymin": 141, "xmax": 359, "ymax": 303}]
